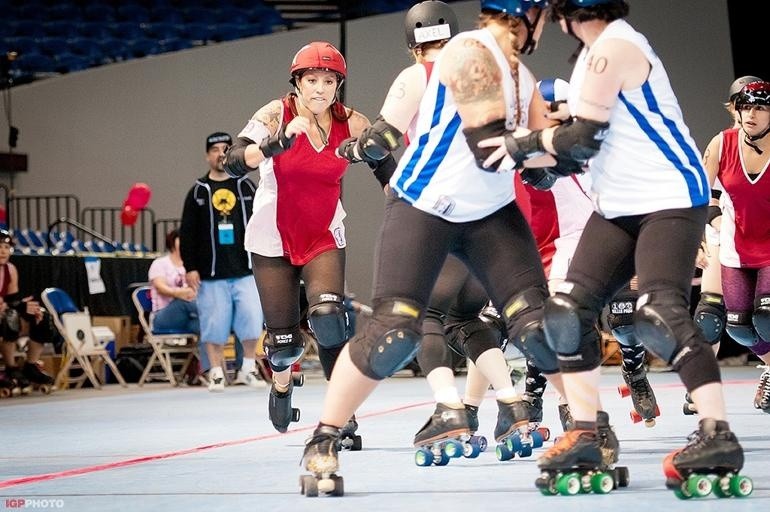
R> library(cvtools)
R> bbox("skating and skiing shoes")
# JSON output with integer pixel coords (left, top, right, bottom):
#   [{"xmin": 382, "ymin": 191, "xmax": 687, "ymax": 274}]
[
  {"xmin": 0, "ymin": 359, "xmax": 57, "ymax": 398},
  {"xmin": 299, "ymin": 412, "xmax": 363, "ymax": 497},
  {"xmin": 268, "ymin": 378, "xmax": 300, "ymax": 434},
  {"xmin": 413, "ymin": 401, "xmax": 487, "ymax": 467},
  {"xmin": 535, "ymin": 401, "xmax": 631, "ymax": 495},
  {"xmin": 491, "ymin": 392, "xmax": 551, "ymax": 462},
  {"xmin": 661, "ymin": 418, "xmax": 753, "ymax": 501},
  {"xmin": 754, "ymin": 369, "xmax": 770, "ymax": 414},
  {"xmin": 618, "ymin": 363, "xmax": 662, "ymax": 428}
]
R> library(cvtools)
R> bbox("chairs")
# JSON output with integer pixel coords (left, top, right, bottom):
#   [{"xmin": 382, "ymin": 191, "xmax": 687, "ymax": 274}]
[
  {"xmin": 1, "ymin": 224, "xmax": 157, "ymax": 258},
  {"xmin": 40, "ymin": 287, "xmax": 126, "ymax": 390},
  {"xmin": 132, "ymin": 286, "xmax": 198, "ymax": 385}
]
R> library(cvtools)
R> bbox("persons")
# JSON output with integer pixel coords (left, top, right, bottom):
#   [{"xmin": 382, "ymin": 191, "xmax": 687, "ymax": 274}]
[
  {"xmin": 220, "ymin": 38, "xmax": 400, "ymax": 445},
  {"xmin": 144, "ymin": 230, "xmax": 246, "ymax": 387},
  {"xmin": 177, "ymin": 127, "xmax": 274, "ymax": 391},
  {"xmin": 294, "ymin": 1, "xmax": 770, "ymax": 497},
  {"xmin": 0, "ymin": 227, "xmax": 57, "ymax": 389}
]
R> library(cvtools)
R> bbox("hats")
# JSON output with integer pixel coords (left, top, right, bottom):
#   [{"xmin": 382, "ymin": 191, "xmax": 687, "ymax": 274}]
[{"xmin": 206, "ymin": 131, "xmax": 234, "ymax": 151}]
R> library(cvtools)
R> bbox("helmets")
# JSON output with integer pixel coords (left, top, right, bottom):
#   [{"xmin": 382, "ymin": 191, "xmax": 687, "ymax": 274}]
[
  {"xmin": 729, "ymin": 76, "xmax": 770, "ymax": 111},
  {"xmin": 478, "ymin": 0, "xmax": 553, "ymax": 18},
  {"xmin": 0, "ymin": 228, "xmax": 16, "ymax": 247},
  {"xmin": 290, "ymin": 41, "xmax": 348, "ymax": 85},
  {"xmin": 403, "ymin": 0, "xmax": 460, "ymax": 51}
]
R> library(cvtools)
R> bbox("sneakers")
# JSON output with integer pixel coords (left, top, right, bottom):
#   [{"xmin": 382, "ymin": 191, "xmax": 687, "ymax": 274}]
[
  {"xmin": 233, "ymin": 370, "xmax": 267, "ymax": 388},
  {"xmin": 187, "ymin": 375, "xmax": 209, "ymax": 387},
  {"xmin": 208, "ymin": 376, "xmax": 225, "ymax": 393}
]
